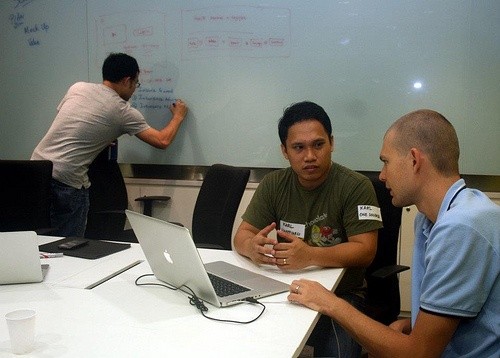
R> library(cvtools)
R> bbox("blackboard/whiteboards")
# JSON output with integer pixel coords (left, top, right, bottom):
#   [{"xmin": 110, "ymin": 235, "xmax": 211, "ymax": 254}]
[{"xmin": 0, "ymin": 0, "xmax": 500, "ymax": 193}]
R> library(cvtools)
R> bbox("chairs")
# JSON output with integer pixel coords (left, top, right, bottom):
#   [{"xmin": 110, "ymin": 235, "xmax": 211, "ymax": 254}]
[
  {"xmin": 0, "ymin": 159, "xmax": 62, "ymax": 236},
  {"xmin": 364, "ymin": 171, "xmax": 411, "ymax": 327},
  {"xmin": 192, "ymin": 163, "xmax": 249, "ymax": 250},
  {"xmin": 83, "ymin": 159, "xmax": 184, "ymax": 244}
]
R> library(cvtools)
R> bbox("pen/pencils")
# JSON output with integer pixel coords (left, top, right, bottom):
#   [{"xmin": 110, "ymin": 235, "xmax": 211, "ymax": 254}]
[{"xmin": 173, "ymin": 103, "xmax": 176, "ymax": 107}]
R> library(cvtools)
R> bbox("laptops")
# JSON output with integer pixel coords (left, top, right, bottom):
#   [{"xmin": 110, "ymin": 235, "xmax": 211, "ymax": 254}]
[
  {"xmin": 125, "ymin": 209, "xmax": 291, "ymax": 308},
  {"xmin": 0, "ymin": 231, "xmax": 49, "ymax": 285}
]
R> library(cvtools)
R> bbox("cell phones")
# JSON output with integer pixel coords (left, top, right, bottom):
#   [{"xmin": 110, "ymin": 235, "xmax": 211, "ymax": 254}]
[{"xmin": 58, "ymin": 239, "xmax": 90, "ymax": 250}]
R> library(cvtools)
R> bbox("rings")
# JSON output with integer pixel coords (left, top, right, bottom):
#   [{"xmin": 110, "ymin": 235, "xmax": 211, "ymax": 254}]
[
  {"xmin": 295, "ymin": 286, "xmax": 300, "ymax": 293},
  {"xmin": 284, "ymin": 259, "xmax": 286, "ymax": 264}
]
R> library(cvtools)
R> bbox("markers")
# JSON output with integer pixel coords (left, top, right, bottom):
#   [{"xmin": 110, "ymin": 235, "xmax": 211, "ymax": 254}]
[{"xmin": 40, "ymin": 253, "xmax": 63, "ymax": 257}]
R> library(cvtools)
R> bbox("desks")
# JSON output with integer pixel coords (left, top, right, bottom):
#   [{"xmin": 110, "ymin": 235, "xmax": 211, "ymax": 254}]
[{"xmin": 0, "ymin": 239, "xmax": 348, "ymax": 358}]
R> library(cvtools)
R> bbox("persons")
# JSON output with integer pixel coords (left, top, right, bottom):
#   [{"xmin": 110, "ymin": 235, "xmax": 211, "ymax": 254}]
[
  {"xmin": 233, "ymin": 102, "xmax": 384, "ymax": 358},
  {"xmin": 288, "ymin": 108, "xmax": 500, "ymax": 358},
  {"xmin": 31, "ymin": 52, "xmax": 188, "ymax": 239}
]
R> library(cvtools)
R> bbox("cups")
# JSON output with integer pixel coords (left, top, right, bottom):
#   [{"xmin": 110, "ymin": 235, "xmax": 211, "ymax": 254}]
[{"xmin": 5, "ymin": 308, "xmax": 36, "ymax": 355}]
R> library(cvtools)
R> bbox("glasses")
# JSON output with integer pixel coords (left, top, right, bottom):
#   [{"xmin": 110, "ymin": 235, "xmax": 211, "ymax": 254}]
[{"xmin": 136, "ymin": 82, "xmax": 140, "ymax": 88}]
